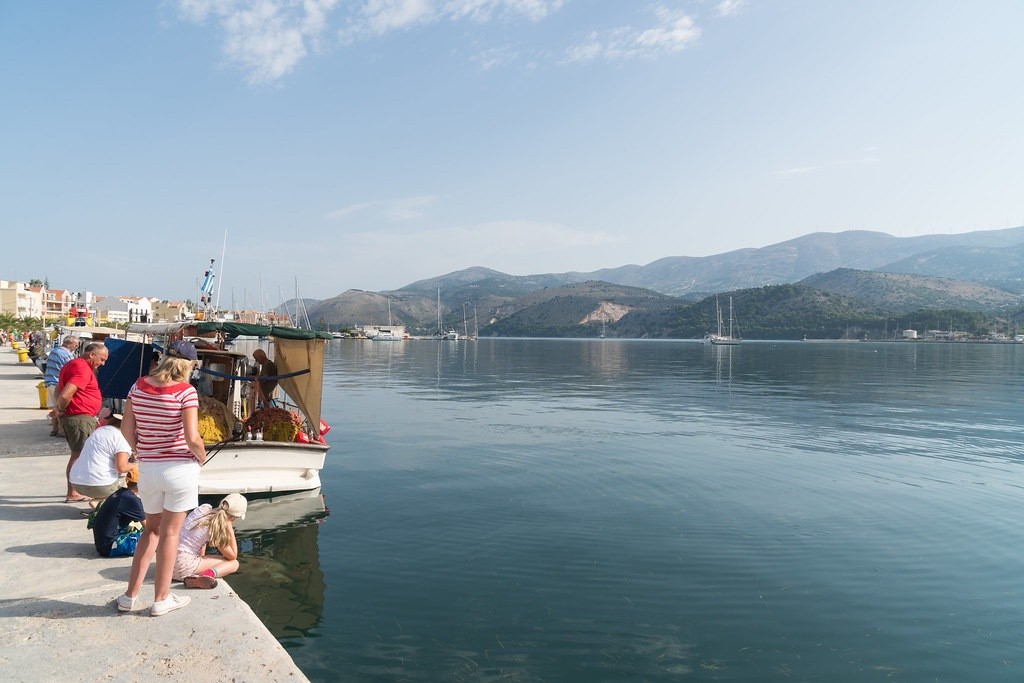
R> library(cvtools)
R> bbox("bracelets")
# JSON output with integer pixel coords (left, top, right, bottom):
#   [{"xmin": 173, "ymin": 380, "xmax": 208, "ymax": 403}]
[{"xmin": 57, "ymin": 406, "xmax": 67, "ymax": 412}]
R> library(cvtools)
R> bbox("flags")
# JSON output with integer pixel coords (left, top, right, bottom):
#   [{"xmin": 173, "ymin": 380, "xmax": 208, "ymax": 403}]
[{"xmin": 200, "ymin": 263, "xmax": 215, "ymax": 296}]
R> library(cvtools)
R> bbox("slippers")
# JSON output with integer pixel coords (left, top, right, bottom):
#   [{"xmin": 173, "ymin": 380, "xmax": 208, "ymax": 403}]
[
  {"xmin": 80, "ymin": 511, "xmax": 90, "ymax": 516},
  {"xmin": 65, "ymin": 496, "xmax": 93, "ymax": 503}
]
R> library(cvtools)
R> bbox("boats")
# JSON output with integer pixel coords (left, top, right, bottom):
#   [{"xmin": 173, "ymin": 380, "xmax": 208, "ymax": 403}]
[{"xmin": 26, "ymin": 318, "xmax": 332, "ymax": 498}]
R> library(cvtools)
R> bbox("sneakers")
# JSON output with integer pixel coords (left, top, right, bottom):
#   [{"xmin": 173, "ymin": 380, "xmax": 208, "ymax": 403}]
[
  {"xmin": 151, "ymin": 592, "xmax": 191, "ymax": 616},
  {"xmin": 116, "ymin": 593, "xmax": 138, "ymax": 611}
]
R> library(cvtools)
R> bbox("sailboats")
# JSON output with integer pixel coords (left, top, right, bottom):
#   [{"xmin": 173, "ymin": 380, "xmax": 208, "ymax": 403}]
[
  {"xmin": 700, "ymin": 293, "xmax": 743, "ymax": 345},
  {"xmin": 371, "ymin": 297, "xmax": 404, "ymax": 341},
  {"xmin": 599, "ymin": 313, "xmax": 607, "ymax": 338},
  {"xmin": 434, "ymin": 286, "xmax": 479, "ymax": 340}
]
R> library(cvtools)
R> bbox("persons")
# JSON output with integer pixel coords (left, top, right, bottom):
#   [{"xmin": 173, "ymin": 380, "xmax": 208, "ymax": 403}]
[
  {"xmin": 171, "ymin": 492, "xmax": 247, "ymax": 589},
  {"xmin": 0, "ymin": 328, "xmax": 35, "ymax": 348},
  {"xmin": 42, "ymin": 336, "xmax": 79, "ymax": 437},
  {"xmin": 49, "ymin": 343, "xmax": 108, "ymax": 503},
  {"xmin": 252, "ymin": 349, "xmax": 278, "ymax": 396},
  {"xmin": 93, "ymin": 464, "xmax": 146, "ymax": 558},
  {"xmin": 68, "ymin": 413, "xmax": 138, "ymax": 516},
  {"xmin": 116, "ymin": 341, "xmax": 207, "ymax": 616}
]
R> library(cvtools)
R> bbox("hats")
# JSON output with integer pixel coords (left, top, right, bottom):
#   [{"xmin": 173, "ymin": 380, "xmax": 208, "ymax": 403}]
[
  {"xmin": 113, "ymin": 413, "xmax": 123, "ymax": 421},
  {"xmin": 126, "ymin": 466, "xmax": 138, "ymax": 484},
  {"xmin": 166, "ymin": 340, "xmax": 198, "ymax": 360},
  {"xmin": 221, "ymin": 493, "xmax": 248, "ymax": 520}
]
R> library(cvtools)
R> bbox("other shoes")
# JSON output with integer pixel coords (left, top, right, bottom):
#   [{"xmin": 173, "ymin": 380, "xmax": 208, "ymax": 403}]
[
  {"xmin": 50, "ymin": 431, "xmax": 56, "ymax": 436},
  {"xmin": 183, "ymin": 574, "xmax": 218, "ymax": 589},
  {"xmin": 57, "ymin": 433, "xmax": 65, "ymax": 437}
]
